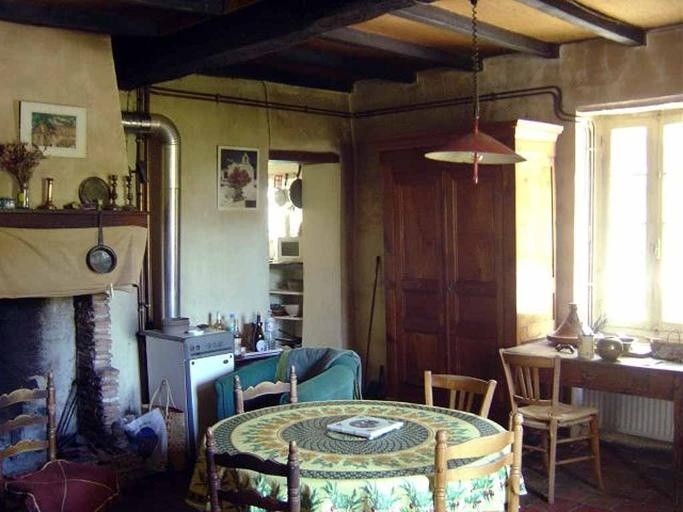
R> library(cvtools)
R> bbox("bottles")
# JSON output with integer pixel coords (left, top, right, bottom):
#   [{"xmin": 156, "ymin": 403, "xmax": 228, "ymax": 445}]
[{"xmin": 214, "ymin": 308, "xmax": 277, "ymax": 353}]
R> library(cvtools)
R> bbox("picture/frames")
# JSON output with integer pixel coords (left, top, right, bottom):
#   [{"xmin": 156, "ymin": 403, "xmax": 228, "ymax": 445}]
[
  {"xmin": 215, "ymin": 144, "xmax": 261, "ymax": 212},
  {"xmin": 16, "ymin": 98, "xmax": 89, "ymax": 160}
]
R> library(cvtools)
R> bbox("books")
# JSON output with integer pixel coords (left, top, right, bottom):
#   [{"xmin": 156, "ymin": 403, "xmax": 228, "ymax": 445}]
[{"xmin": 326, "ymin": 415, "xmax": 404, "ymax": 441}]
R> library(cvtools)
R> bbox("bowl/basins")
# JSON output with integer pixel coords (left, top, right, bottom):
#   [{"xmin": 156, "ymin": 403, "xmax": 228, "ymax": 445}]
[
  {"xmin": 287, "ymin": 279, "xmax": 302, "ymax": 292},
  {"xmin": 283, "ymin": 304, "xmax": 302, "ymax": 318}
]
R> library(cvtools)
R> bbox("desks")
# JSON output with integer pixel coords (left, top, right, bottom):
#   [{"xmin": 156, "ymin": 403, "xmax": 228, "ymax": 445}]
[
  {"xmin": 502, "ymin": 332, "xmax": 683, "ymax": 508},
  {"xmin": 200, "ymin": 399, "xmax": 512, "ymax": 512}
]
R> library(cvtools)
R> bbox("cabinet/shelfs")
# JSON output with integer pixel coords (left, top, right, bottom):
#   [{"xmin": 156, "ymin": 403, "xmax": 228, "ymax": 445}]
[
  {"xmin": 366, "ymin": 118, "xmax": 570, "ymax": 429},
  {"xmin": 269, "ymin": 262, "xmax": 303, "ymax": 351}
]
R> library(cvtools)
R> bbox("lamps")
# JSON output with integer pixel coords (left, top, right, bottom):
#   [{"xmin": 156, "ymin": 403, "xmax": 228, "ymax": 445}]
[{"xmin": 422, "ymin": 6, "xmax": 527, "ymax": 186}]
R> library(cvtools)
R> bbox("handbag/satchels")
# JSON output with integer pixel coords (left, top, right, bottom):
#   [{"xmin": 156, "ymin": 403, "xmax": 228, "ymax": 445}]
[{"xmin": 124, "ymin": 378, "xmax": 186, "ymax": 478}]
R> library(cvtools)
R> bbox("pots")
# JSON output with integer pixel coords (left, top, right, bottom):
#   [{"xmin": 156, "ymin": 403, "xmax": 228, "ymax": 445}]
[
  {"xmin": 289, "ymin": 163, "xmax": 302, "ymax": 210},
  {"xmin": 88, "ymin": 212, "xmax": 118, "ymax": 274}
]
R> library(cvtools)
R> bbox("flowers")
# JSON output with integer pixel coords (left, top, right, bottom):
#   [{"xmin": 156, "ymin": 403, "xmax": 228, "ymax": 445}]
[{"xmin": 0, "ymin": 137, "xmax": 48, "ymax": 185}]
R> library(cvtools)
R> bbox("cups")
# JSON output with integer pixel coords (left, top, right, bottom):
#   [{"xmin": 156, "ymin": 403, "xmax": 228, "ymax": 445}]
[{"xmin": 578, "ymin": 335, "xmax": 594, "ymax": 360}]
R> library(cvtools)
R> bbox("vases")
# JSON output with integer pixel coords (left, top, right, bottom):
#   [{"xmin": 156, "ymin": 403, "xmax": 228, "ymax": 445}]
[{"xmin": 15, "ymin": 188, "xmax": 31, "ymax": 208}]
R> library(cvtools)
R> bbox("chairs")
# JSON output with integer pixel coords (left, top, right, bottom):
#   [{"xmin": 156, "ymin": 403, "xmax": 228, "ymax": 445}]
[
  {"xmin": 0, "ymin": 369, "xmax": 122, "ymax": 510},
  {"xmin": 421, "ymin": 347, "xmax": 610, "ymax": 511},
  {"xmin": 201, "ymin": 343, "xmax": 362, "ymax": 512}
]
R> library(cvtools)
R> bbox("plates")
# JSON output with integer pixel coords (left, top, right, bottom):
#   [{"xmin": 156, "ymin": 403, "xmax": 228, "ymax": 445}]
[{"xmin": 79, "ymin": 177, "xmax": 111, "ymax": 208}]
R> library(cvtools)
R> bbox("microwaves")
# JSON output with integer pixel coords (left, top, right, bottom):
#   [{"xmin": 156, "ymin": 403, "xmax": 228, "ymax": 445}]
[{"xmin": 278, "ymin": 238, "xmax": 302, "ymax": 260}]
